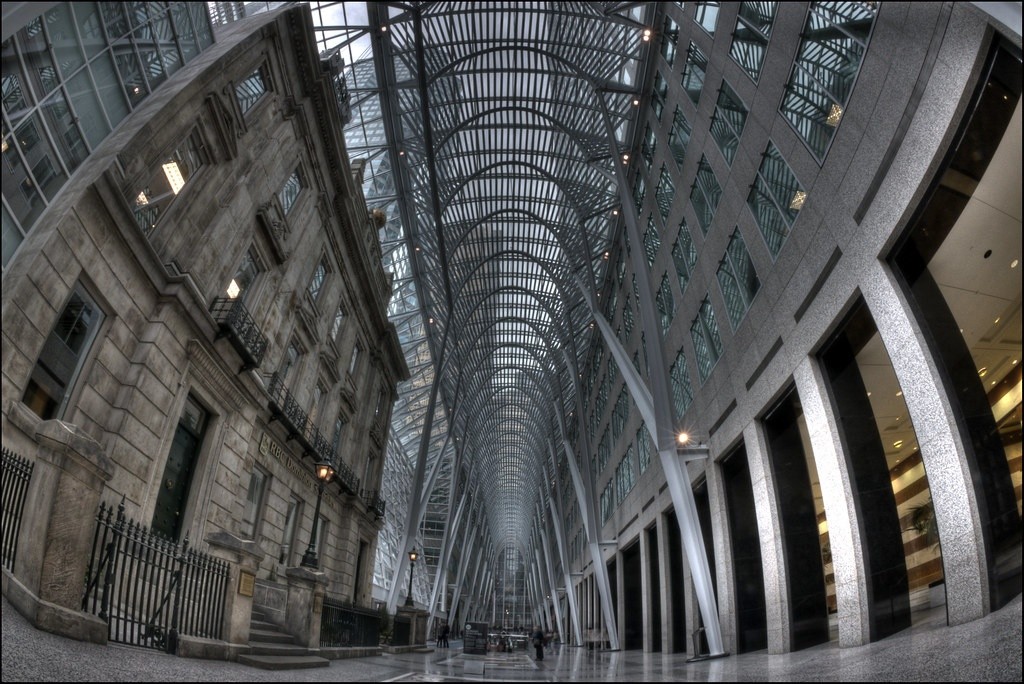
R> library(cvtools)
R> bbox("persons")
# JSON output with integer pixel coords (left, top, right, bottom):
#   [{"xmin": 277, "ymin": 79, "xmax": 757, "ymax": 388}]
[
  {"xmin": 513, "ymin": 624, "xmax": 536, "ymax": 637},
  {"xmin": 492, "ymin": 624, "xmax": 503, "ymax": 631},
  {"xmin": 532, "ymin": 626, "xmax": 548, "ymax": 662},
  {"xmin": 436, "ymin": 621, "xmax": 450, "ymax": 648}
]
